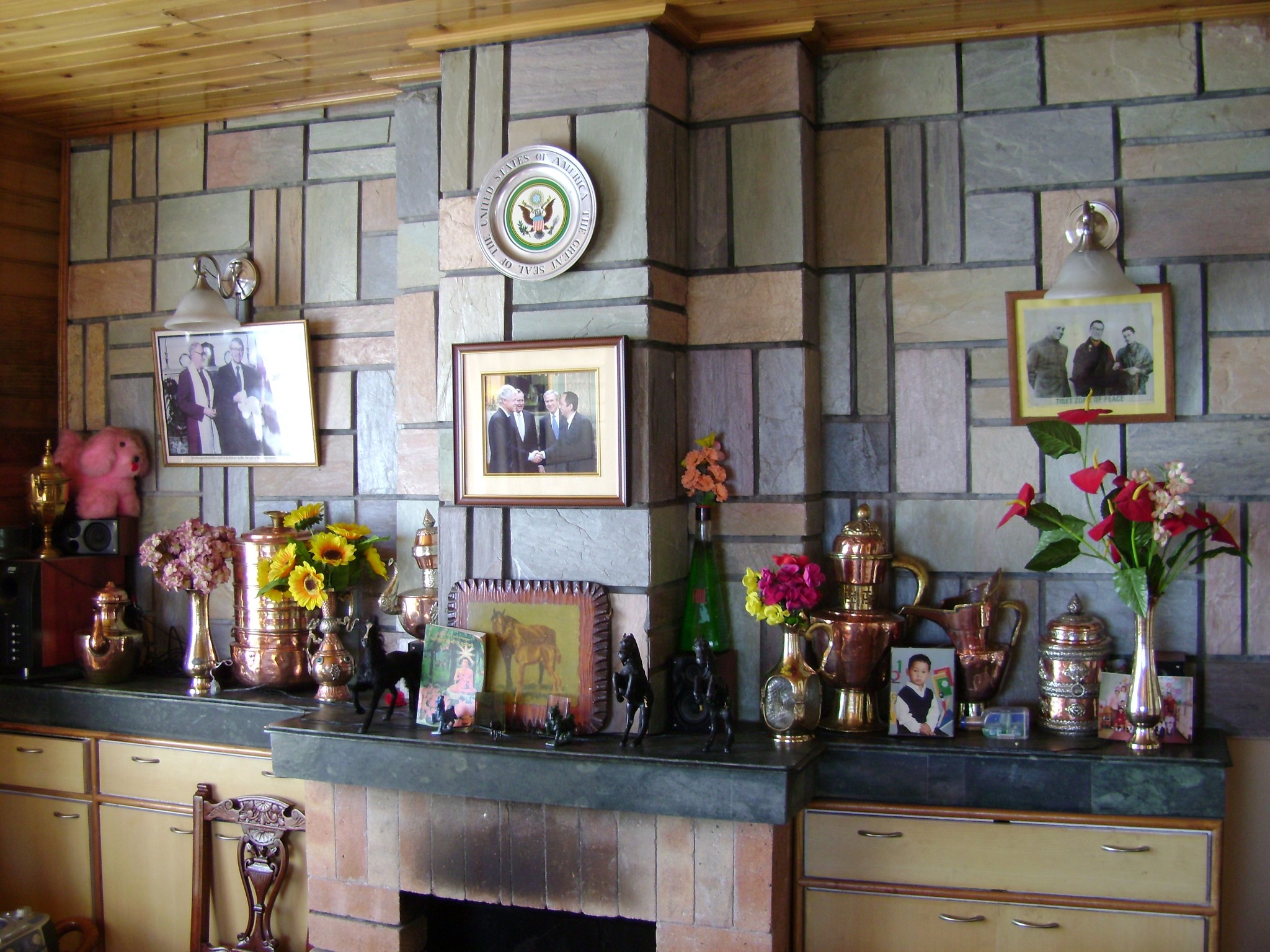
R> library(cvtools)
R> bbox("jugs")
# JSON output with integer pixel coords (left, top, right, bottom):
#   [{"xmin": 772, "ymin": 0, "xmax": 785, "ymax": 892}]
[
  {"xmin": 809, "ymin": 499, "xmax": 928, "ymax": 735},
  {"xmin": 900, "ymin": 565, "xmax": 1025, "ymax": 731}
]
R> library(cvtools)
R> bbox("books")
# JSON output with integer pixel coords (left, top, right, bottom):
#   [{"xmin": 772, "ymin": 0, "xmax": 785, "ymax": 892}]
[{"xmin": 416, "ymin": 624, "xmax": 486, "ymax": 732}]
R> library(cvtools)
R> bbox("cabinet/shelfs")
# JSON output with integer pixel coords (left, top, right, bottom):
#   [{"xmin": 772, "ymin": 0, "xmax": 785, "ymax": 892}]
[
  {"xmin": 0, "ymin": 721, "xmax": 307, "ymax": 948},
  {"xmin": 791, "ymin": 798, "xmax": 1222, "ymax": 952}
]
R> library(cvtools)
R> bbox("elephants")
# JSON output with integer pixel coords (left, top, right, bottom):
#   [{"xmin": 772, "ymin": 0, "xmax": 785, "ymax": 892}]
[{"xmin": 490, "ymin": 720, "xmax": 503, "ymax": 740}]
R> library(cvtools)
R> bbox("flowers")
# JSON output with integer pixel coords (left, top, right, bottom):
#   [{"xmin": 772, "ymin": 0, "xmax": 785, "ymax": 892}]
[
  {"xmin": 138, "ymin": 518, "xmax": 237, "ymax": 592},
  {"xmin": 680, "ymin": 434, "xmax": 731, "ymax": 509},
  {"xmin": 743, "ymin": 555, "xmax": 824, "ymax": 631},
  {"xmin": 258, "ymin": 501, "xmax": 389, "ymax": 610},
  {"xmin": 998, "ymin": 390, "xmax": 1254, "ymax": 620}
]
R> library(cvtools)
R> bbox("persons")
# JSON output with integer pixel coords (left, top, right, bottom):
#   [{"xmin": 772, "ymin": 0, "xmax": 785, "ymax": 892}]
[
  {"xmin": 1027, "ymin": 320, "xmax": 1154, "ymax": 397},
  {"xmin": 449, "ymin": 658, "xmax": 475, "ymax": 694},
  {"xmin": 176, "ymin": 337, "xmax": 260, "ymax": 454},
  {"xmin": 489, "ymin": 384, "xmax": 596, "ymax": 473},
  {"xmin": 1165, "ymin": 691, "xmax": 1177, "ymax": 735},
  {"xmin": 895, "ymin": 654, "xmax": 938, "ymax": 736},
  {"xmin": 1110, "ymin": 686, "xmax": 1134, "ymax": 734}
]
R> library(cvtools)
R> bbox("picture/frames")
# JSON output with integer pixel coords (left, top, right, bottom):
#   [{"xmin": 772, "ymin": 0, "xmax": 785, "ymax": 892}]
[
  {"xmin": 148, "ymin": 320, "xmax": 324, "ymax": 469},
  {"xmin": 451, "ymin": 332, "xmax": 629, "ymax": 510},
  {"xmin": 1005, "ymin": 281, "xmax": 1178, "ymax": 424},
  {"xmin": 883, "ymin": 640, "xmax": 959, "ymax": 742}
]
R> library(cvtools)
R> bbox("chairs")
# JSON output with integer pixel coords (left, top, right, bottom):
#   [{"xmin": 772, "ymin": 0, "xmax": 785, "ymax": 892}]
[{"xmin": 188, "ymin": 779, "xmax": 306, "ymax": 952}]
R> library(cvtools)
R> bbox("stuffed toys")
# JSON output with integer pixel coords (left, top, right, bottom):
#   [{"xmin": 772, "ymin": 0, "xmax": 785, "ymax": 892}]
[{"xmin": 54, "ymin": 426, "xmax": 149, "ymax": 518}]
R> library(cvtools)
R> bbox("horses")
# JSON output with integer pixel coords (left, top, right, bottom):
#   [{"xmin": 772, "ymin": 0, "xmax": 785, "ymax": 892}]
[
  {"xmin": 352, "ymin": 621, "xmax": 423, "ymax": 736},
  {"xmin": 491, "ymin": 607, "xmax": 557, "ymax": 697},
  {"xmin": 692, "ymin": 636, "xmax": 739, "ymax": 753},
  {"xmin": 613, "ymin": 633, "xmax": 654, "ymax": 748},
  {"xmin": 501, "ymin": 621, "xmax": 562, "ymax": 704}
]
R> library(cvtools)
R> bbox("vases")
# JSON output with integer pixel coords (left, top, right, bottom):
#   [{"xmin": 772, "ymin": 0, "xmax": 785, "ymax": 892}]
[
  {"xmin": 681, "ymin": 505, "xmax": 733, "ymax": 655},
  {"xmin": 1126, "ymin": 591, "xmax": 1167, "ymax": 753},
  {"xmin": 185, "ymin": 586, "xmax": 222, "ymax": 696},
  {"xmin": 302, "ymin": 589, "xmax": 356, "ymax": 702},
  {"xmin": 761, "ymin": 612, "xmax": 833, "ymax": 744}
]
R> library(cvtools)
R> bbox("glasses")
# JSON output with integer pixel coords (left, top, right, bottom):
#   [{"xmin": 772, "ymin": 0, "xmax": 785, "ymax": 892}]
[
  {"xmin": 231, "ymin": 348, "xmax": 245, "ymax": 351},
  {"xmin": 194, "ymin": 351, "xmax": 206, "ymax": 355}
]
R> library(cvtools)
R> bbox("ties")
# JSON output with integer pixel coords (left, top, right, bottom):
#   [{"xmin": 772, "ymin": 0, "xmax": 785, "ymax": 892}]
[
  {"xmin": 518, "ymin": 413, "xmax": 525, "ymax": 442},
  {"xmin": 237, "ymin": 366, "xmax": 242, "ymax": 390},
  {"xmin": 552, "ymin": 415, "xmax": 559, "ymax": 440},
  {"xmin": 565, "ymin": 421, "xmax": 568, "ymax": 430}
]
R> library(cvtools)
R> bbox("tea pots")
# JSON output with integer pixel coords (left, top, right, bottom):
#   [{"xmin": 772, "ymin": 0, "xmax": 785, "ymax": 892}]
[{"xmin": 73, "ymin": 581, "xmax": 144, "ymax": 686}]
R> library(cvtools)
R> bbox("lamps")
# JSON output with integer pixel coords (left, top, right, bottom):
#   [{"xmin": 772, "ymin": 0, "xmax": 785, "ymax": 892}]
[
  {"xmin": 163, "ymin": 250, "xmax": 261, "ymax": 333},
  {"xmin": 1041, "ymin": 200, "xmax": 1143, "ymax": 300}
]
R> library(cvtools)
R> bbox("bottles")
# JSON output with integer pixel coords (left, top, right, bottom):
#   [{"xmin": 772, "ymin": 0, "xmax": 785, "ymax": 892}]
[{"xmin": 1036, "ymin": 593, "xmax": 1111, "ymax": 736}]
